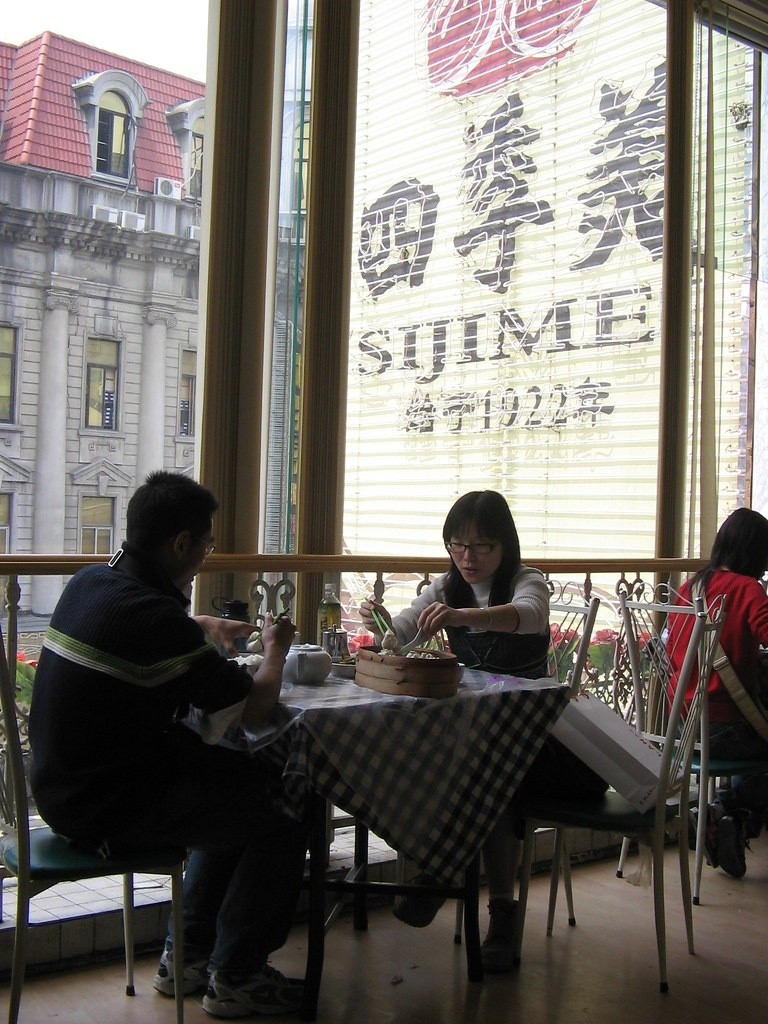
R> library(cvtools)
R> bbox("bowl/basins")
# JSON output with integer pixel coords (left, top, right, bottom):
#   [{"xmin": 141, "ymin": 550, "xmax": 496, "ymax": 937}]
[
  {"xmin": 328, "ymin": 656, "xmax": 356, "ymax": 678},
  {"xmin": 224, "ymin": 658, "xmax": 259, "ymax": 676}
]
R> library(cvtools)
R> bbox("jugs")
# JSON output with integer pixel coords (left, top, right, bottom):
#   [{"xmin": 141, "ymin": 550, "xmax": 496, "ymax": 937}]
[{"xmin": 323, "ymin": 623, "xmax": 351, "ymax": 661}]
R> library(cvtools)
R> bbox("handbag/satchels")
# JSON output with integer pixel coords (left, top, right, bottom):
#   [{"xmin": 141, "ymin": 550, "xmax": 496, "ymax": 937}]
[{"xmin": 552, "ymin": 690, "xmax": 684, "ymax": 812}]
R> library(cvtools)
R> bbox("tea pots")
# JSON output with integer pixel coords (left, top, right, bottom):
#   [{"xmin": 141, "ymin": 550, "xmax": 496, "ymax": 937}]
[{"xmin": 282, "ymin": 643, "xmax": 333, "ymax": 685}]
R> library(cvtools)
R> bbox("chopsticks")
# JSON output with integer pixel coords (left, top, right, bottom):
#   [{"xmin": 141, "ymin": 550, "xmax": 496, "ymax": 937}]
[
  {"xmin": 249, "ymin": 608, "xmax": 290, "ymax": 641},
  {"xmin": 364, "ymin": 597, "xmax": 395, "ymax": 637}
]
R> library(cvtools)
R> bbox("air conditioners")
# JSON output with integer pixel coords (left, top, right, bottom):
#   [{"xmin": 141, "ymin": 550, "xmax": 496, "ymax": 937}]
[
  {"xmin": 154, "ymin": 177, "xmax": 181, "ymax": 200},
  {"xmin": 187, "ymin": 225, "xmax": 200, "ymax": 240},
  {"xmin": 88, "ymin": 204, "xmax": 146, "ymax": 231}
]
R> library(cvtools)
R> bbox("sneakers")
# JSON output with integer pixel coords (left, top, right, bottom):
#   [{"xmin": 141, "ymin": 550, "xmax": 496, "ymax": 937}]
[
  {"xmin": 201, "ymin": 967, "xmax": 307, "ymax": 1017},
  {"xmin": 155, "ymin": 937, "xmax": 215, "ymax": 997},
  {"xmin": 718, "ymin": 814, "xmax": 753, "ymax": 878},
  {"xmin": 690, "ymin": 807, "xmax": 721, "ymax": 868}
]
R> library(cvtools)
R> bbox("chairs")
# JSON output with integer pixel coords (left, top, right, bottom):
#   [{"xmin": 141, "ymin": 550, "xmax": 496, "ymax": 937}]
[
  {"xmin": 0, "ymin": 624, "xmax": 184, "ymax": 1024},
  {"xmin": 440, "ymin": 580, "xmax": 768, "ymax": 993}
]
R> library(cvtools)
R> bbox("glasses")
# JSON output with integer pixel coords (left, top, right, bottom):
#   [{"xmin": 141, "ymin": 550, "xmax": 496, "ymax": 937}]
[
  {"xmin": 180, "ymin": 529, "xmax": 215, "ymax": 557},
  {"xmin": 445, "ymin": 541, "xmax": 506, "ymax": 554}
]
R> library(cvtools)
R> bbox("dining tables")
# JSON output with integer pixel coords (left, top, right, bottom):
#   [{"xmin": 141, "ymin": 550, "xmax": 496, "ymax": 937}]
[{"xmin": 186, "ymin": 667, "xmax": 572, "ymax": 1003}]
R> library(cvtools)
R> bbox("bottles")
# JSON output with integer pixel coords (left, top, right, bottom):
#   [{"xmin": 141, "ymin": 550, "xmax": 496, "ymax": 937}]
[
  {"xmin": 317, "ymin": 583, "xmax": 341, "ymax": 648},
  {"xmin": 212, "ymin": 596, "xmax": 250, "ymax": 657}
]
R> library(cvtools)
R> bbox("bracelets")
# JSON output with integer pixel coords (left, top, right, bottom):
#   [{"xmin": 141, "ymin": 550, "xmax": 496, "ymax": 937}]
[{"xmin": 475, "ymin": 608, "xmax": 492, "ymax": 631}]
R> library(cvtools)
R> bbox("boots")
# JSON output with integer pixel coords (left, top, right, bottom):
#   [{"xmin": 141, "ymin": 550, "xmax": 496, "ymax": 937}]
[
  {"xmin": 479, "ymin": 898, "xmax": 521, "ymax": 977},
  {"xmin": 390, "ymin": 871, "xmax": 446, "ymax": 928}
]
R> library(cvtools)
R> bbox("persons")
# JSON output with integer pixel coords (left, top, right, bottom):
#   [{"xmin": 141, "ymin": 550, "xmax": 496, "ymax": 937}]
[
  {"xmin": 662, "ymin": 508, "xmax": 768, "ymax": 878},
  {"xmin": 28, "ymin": 471, "xmax": 305, "ymax": 1019},
  {"xmin": 360, "ymin": 490, "xmax": 550, "ymax": 975}
]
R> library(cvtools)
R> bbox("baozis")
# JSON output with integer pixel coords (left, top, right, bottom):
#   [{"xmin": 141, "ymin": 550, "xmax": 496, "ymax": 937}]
[
  {"xmin": 226, "ymin": 631, "xmax": 264, "ymax": 666},
  {"xmin": 377, "ymin": 630, "xmax": 436, "ymax": 659}
]
